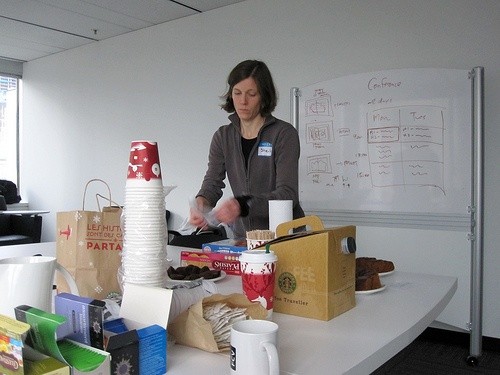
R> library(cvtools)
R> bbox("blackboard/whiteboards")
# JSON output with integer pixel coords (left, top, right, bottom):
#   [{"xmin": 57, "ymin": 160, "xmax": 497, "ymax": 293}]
[{"xmin": 291, "ymin": 68, "xmax": 477, "ymax": 229}]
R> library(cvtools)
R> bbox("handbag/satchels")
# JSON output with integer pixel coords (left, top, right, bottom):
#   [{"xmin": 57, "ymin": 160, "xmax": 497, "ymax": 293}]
[
  {"xmin": 168, "ymin": 224, "xmax": 228, "ymax": 249},
  {"xmin": 54, "ymin": 179, "xmax": 124, "ymax": 300}
]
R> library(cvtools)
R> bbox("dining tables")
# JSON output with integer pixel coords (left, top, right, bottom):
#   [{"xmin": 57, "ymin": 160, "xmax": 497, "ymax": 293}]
[{"xmin": 0, "ymin": 242, "xmax": 458, "ymax": 375}]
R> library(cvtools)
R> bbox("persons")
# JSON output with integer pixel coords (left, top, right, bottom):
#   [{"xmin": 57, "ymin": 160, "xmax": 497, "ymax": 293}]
[{"xmin": 191, "ymin": 60, "xmax": 306, "ymax": 242}]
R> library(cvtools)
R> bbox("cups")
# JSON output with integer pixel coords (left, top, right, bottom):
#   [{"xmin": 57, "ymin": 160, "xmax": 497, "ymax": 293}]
[
  {"xmin": 239, "ymin": 250, "xmax": 278, "ymax": 319},
  {"xmin": 246, "ymin": 239, "xmax": 274, "ymax": 251},
  {"xmin": 230, "ymin": 319, "xmax": 279, "ymax": 375},
  {"xmin": 0, "ymin": 256, "xmax": 57, "ymax": 320},
  {"xmin": 122, "ymin": 140, "xmax": 168, "ymax": 288},
  {"xmin": 268, "ymin": 200, "xmax": 293, "ymax": 237}
]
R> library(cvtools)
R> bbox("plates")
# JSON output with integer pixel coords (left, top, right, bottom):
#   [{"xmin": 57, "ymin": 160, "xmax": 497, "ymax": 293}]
[
  {"xmin": 378, "ymin": 266, "xmax": 396, "ymax": 276},
  {"xmin": 355, "ymin": 284, "xmax": 386, "ymax": 294},
  {"xmin": 168, "ymin": 267, "xmax": 227, "ymax": 284}
]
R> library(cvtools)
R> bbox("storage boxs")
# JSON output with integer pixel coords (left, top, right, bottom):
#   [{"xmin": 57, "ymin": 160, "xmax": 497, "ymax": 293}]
[
  {"xmin": 0, "ymin": 283, "xmax": 174, "ymax": 375},
  {"xmin": 181, "ymin": 251, "xmax": 242, "ymax": 276},
  {"xmin": 254, "ymin": 215, "xmax": 357, "ymax": 321},
  {"xmin": 202, "ymin": 238, "xmax": 247, "ymax": 254}
]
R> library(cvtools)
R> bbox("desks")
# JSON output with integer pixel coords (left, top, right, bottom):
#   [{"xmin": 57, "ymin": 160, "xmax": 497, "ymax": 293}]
[{"xmin": 0, "ymin": 210, "xmax": 50, "ymax": 245}]
